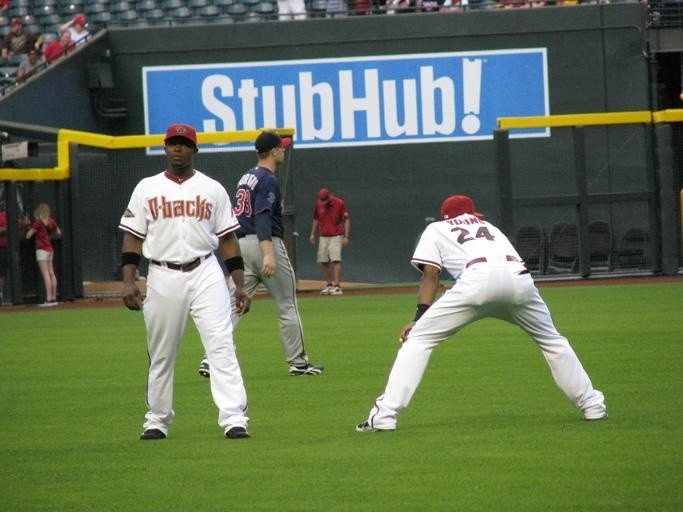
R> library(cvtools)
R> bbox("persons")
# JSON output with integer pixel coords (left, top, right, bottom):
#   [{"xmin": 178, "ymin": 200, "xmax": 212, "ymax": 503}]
[
  {"xmin": 277, "ymin": 0, "xmax": 307, "ymax": 20},
  {"xmin": 26, "ymin": 202, "xmax": 62, "ymax": 306},
  {"xmin": 199, "ymin": 126, "xmax": 325, "ymax": 376},
  {"xmin": 0, "ymin": 200, "xmax": 25, "ymax": 301},
  {"xmin": 356, "ymin": 194, "xmax": 608, "ymax": 433},
  {"xmin": 327, "ymin": 0, "xmax": 470, "ymax": 20},
  {"xmin": 117, "ymin": 122, "xmax": 254, "ymax": 440},
  {"xmin": 308, "ymin": 186, "xmax": 350, "ymax": 295},
  {"xmin": 1, "ymin": 0, "xmax": 85, "ymax": 82},
  {"xmin": 483, "ymin": 0, "xmax": 582, "ymax": 9}
]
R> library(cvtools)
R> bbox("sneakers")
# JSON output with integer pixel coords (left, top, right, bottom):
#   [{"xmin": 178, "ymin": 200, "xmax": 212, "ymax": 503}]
[
  {"xmin": 329, "ymin": 287, "xmax": 343, "ymax": 294},
  {"xmin": 320, "ymin": 286, "xmax": 332, "ymax": 295},
  {"xmin": 140, "ymin": 429, "xmax": 165, "ymax": 439},
  {"xmin": 583, "ymin": 407, "xmax": 606, "ymax": 420},
  {"xmin": 226, "ymin": 427, "xmax": 248, "ymax": 438},
  {"xmin": 355, "ymin": 421, "xmax": 376, "ymax": 431},
  {"xmin": 289, "ymin": 364, "xmax": 324, "ymax": 375},
  {"xmin": 199, "ymin": 359, "xmax": 210, "ymax": 377}
]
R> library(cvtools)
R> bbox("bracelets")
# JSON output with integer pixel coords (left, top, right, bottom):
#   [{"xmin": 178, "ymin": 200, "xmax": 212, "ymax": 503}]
[
  {"xmin": 413, "ymin": 303, "xmax": 430, "ymax": 321},
  {"xmin": 121, "ymin": 250, "xmax": 141, "ymax": 266},
  {"xmin": 222, "ymin": 256, "xmax": 245, "ymax": 273}
]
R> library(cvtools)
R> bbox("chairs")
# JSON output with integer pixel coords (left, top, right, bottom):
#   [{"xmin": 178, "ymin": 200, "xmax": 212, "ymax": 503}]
[
  {"xmin": 546, "ymin": 221, "xmax": 567, "ymax": 244},
  {"xmin": 1, "ymin": 2, "xmax": 326, "ymax": 34},
  {"xmin": 546, "ymin": 224, "xmax": 579, "ymax": 272},
  {"xmin": 615, "ymin": 228, "xmax": 649, "ymax": 270},
  {"xmin": 1, "ymin": 34, "xmax": 60, "ymax": 97},
  {"xmin": 511, "ymin": 224, "xmax": 547, "ymax": 274},
  {"xmin": 587, "ymin": 220, "xmax": 615, "ymax": 272}
]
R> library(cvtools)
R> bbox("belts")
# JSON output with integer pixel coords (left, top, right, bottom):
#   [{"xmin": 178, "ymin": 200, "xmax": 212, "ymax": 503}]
[
  {"xmin": 152, "ymin": 252, "xmax": 211, "ymax": 271},
  {"xmin": 466, "ymin": 255, "xmax": 519, "ymax": 268}
]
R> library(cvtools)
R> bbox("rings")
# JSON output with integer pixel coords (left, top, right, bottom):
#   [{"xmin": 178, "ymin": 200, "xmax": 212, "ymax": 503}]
[{"xmin": 398, "ymin": 337, "xmax": 404, "ymax": 342}]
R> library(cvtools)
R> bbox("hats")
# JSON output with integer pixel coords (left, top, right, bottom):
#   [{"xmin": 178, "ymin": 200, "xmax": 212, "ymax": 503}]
[
  {"xmin": 255, "ymin": 130, "xmax": 292, "ymax": 153},
  {"xmin": 319, "ymin": 189, "xmax": 329, "ymax": 205},
  {"xmin": 164, "ymin": 123, "xmax": 196, "ymax": 145},
  {"xmin": 441, "ymin": 196, "xmax": 484, "ymax": 221}
]
